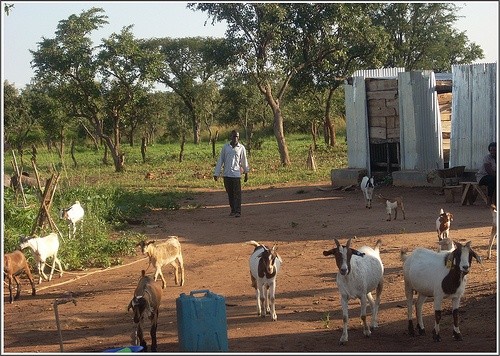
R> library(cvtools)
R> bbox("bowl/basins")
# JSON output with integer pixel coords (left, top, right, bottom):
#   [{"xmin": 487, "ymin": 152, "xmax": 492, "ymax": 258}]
[{"xmin": 105, "ymin": 346, "xmax": 144, "ymax": 353}]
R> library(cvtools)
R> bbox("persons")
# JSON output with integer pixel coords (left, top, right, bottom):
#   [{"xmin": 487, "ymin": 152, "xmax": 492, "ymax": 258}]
[
  {"xmin": 213, "ymin": 130, "xmax": 248, "ymax": 217},
  {"xmin": 475, "ymin": 142, "xmax": 496, "ymax": 209}
]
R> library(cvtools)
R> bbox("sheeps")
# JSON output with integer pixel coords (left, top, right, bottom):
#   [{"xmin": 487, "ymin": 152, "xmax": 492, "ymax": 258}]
[
  {"xmin": 323, "ymin": 237, "xmax": 385, "ymax": 344},
  {"xmin": 4, "ymin": 251, "xmax": 37, "ymax": 302},
  {"xmin": 399, "ymin": 240, "xmax": 481, "ymax": 343},
  {"xmin": 18, "ymin": 234, "xmax": 63, "ymax": 283},
  {"xmin": 247, "ymin": 241, "xmax": 283, "ymax": 321},
  {"xmin": 127, "ymin": 270, "xmax": 162, "ymax": 352},
  {"xmin": 360, "ymin": 176, "xmax": 374, "ymax": 209},
  {"xmin": 377, "ymin": 194, "xmax": 406, "ymax": 221},
  {"xmin": 437, "ymin": 239, "xmax": 456, "ymax": 254},
  {"xmin": 58, "ymin": 201, "xmax": 85, "ymax": 239},
  {"xmin": 435, "ymin": 209, "xmax": 453, "ymax": 242},
  {"xmin": 486, "ymin": 204, "xmax": 497, "ymax": 260},
  {"xmin": 134, "ymin": 236, "xmax": 185, "ymax": 289}
]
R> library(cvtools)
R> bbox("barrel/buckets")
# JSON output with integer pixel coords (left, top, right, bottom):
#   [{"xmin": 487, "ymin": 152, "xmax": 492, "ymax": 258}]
[
  {"xmin": 459, "ymin": 171, "xmax": 478, "ymax": 205},
  {"xmin": 444, "ymin": 185, "xmax": 463, "ymax": 203},
  {"xmin": 176, "ymin": 289, "xmax": 228, "ymax": 352}
]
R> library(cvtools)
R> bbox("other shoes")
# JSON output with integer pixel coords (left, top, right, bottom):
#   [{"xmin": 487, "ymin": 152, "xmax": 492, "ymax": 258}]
[
  {"xmin": 229, "ymin": 211, "xmax": 241, "ymax": 217},
  {"xmin": 488, "ymin": 201, "xmax": 496, "ymax": 208}
]
